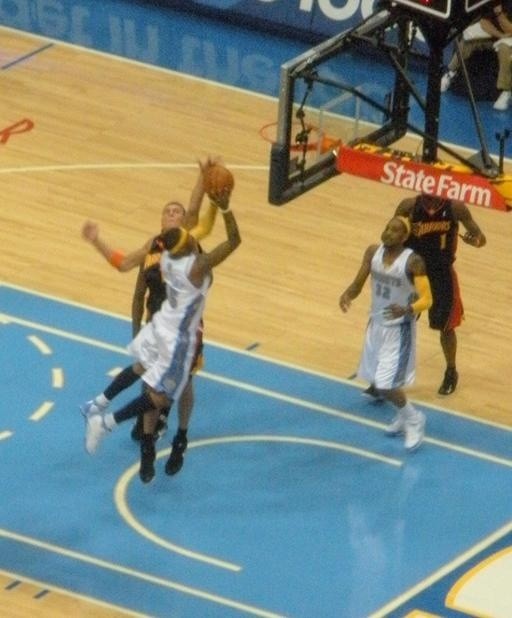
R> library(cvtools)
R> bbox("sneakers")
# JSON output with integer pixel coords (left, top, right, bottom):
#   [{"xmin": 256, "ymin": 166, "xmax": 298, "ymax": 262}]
[
  {"xmin": 438, "ymin": 371, "xmax": 459, "ymax": 395},
  {"xmin": 403, "ymin": 410, "xmax": 427, "ymax": 452},
  {"xmin": 383, "ymin": 416, "xmax": 404, "ymax": 434},
  {"xmin": 131, "ymin": 419, "xmax": 168, "ymax": 484},
  {"xmin": 440, "ymin": 69, "xmax": 457, "ymax": 92},
  {"xmin": 492, "ymin": 90, "xmax": 511, "ymax": 111},
  {"xmin": 165, "ymin": 435, "xmax": 188, "ymax": 476},
  {"xmin": 360, "ymin": 386, "xmax": 380, "ymax": 402},
  {"xmin": 85, "ymin": 414, "xmax": 112, "ymax": 457},
  {"xmin": 78, "ymin": 399, "xmax": 105, "ymax": 417}
]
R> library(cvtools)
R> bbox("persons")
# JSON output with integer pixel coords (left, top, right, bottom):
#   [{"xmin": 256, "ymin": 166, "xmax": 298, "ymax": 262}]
[
  {"xmin": 336, "ymin": 212, "xmax": 436, "ymax": 453},
  {"xmin": 74, "ymin": 183, "xmax": 242, "ymax": 456},
  {"xmin": 438, "ymin": 0, "xmax": 512, "ymax": 111},
  {"xmin": 356, "ymin": 190, "xmax": 487, "ymax": 404},
  {"xmin": 80, "ymin": 151, "xmax": 225, "ymax": 484},
  {"xmin": 128, "ymin": 197, "xmax": 207, "ymax": 445}
]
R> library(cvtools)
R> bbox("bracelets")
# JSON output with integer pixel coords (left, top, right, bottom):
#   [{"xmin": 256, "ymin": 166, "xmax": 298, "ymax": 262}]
[
  {"xmin": 401, "ymin": 304, "xmax": 414, "ymax": 314},
  {"xmin": 219, "ymin": 206, "xmax": 232, "ymax": 214},
  {"xmin": 494, "ymin": 9, "xmax": 504, "ymax": 16},
  {"xmin": 476, "ymin": 240, "xmax": 481, "ymax": 247}
]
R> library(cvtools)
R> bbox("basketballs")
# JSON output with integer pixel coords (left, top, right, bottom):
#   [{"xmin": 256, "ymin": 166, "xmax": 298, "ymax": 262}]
[{"xmin": 202, "ymin": 165, "xmax": 234, "ymax": 196}]
